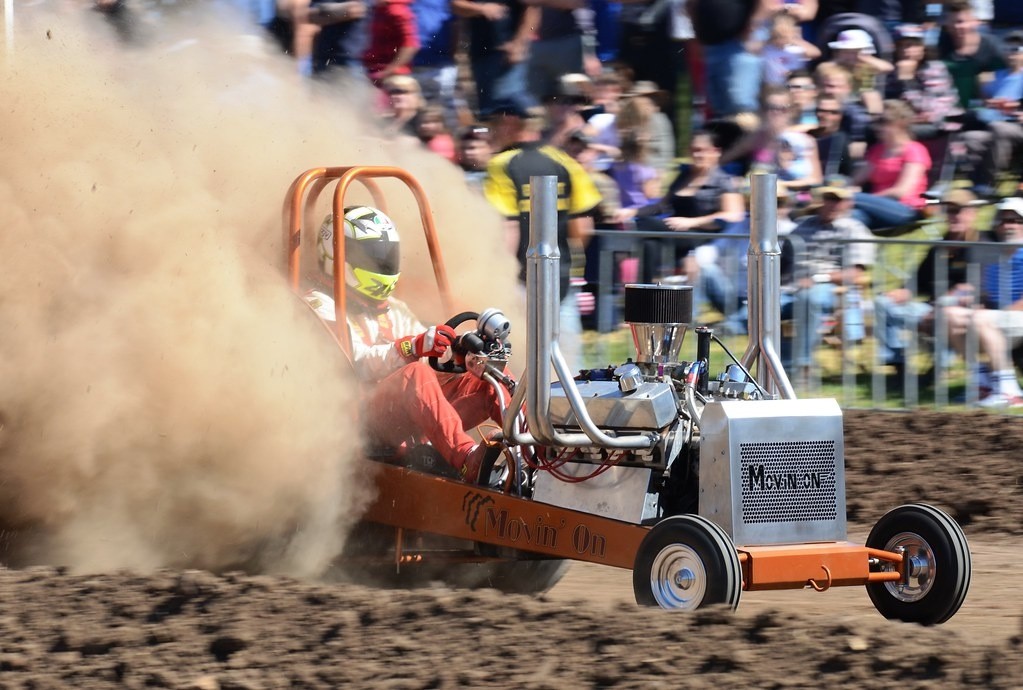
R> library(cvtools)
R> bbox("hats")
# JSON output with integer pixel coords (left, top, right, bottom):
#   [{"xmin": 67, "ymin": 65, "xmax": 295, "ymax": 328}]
[
  {"xmin": 895, "ymin": 22, "xmax": 925, "ymax": 42},
  {"xmin": 475, "ymin": 98, "xmax": 531, "ymax": 121},
  {"xmin": 994, "ymin": 197, "xmax": 1023, "ymax": 217},
  {"xmin": 616, "ymin": 80, "xmax": 664, "ymax": 104},
  {"xmin": 827, "ymin": 29, "xmax": 874, "ymax": 49},
  {"xmin": 810, "ymin": 179, "xmax": 862, "ymax": 199}
]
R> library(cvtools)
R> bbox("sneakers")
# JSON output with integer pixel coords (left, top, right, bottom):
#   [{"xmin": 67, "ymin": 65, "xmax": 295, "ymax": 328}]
[
  {"xmin": 461, "ymin": 429, "xmax": 512, "ymax": 490},
  {"xmin": 972, "ymin": 390, "xmax": 1023, "ymax": 410},
  {"xmin": 955, "ymin": 384, "xmax": 994, "ymax": 402},
  {"xmin": 512, "ymin": 444, "xmax": 540, "ymax": 500}
]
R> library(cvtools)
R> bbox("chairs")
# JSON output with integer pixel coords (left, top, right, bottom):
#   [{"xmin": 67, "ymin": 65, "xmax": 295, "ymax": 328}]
[{"xmin": 869, "ymin": 125, "xmax": 964, "ymax": 281}]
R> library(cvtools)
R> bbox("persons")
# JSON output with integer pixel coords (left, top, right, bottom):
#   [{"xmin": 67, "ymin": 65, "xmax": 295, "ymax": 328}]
[
  {"xmin": 300, "ymin": 206, "xmax": 538, "ymax": 485},
  {"xmin": 203, "ymin": 0, "xmax": 1022, "ymax": 409}
]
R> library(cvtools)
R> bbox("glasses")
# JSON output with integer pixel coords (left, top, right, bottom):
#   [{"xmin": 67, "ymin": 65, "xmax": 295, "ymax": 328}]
[
  {"xmin": 993, "ymin": 216, "xmax": 1023, "ymax": 226},
  {"xmin": 386, "ymin": 89, "xmax": 414, "ymax": 95},
  {"xmin": 1001, "ymin": 46, "xmax": 1023, "ymax": 54},
  {"xmin": 815, "ymin": 107, "xmax": 842, "ymax": 116}
]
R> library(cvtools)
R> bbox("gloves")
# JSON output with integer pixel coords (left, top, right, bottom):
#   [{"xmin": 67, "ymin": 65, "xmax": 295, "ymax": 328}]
[{"xmin": 395, "ymin": 325, "xmax": 456, "ymax": 364}]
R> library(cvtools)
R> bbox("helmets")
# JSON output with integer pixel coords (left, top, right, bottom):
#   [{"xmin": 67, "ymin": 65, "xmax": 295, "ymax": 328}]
[{"xmin": 318, "ymin": 206, "xmax": 401, "ymax": 315}]
